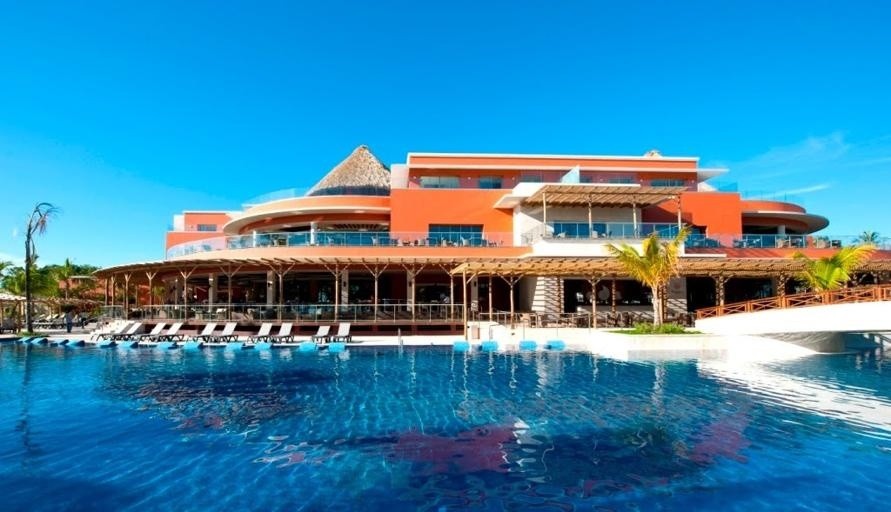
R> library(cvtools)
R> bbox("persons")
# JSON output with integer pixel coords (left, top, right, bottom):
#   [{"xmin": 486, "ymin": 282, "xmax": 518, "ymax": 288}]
[{"xmin": 66, "ymin": 307, "xmax": 74, "ymax": 333}]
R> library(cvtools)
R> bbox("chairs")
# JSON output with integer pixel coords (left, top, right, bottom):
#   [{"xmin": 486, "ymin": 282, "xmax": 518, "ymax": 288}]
[
  {"xmin": 90, "ymin": 320, "xmax": 135, "ymax": 341},
  {"xmin": 21, "ymin": 313, "xmax": 78, "ymax": 329},
  {"xmin": 272, "ymin": 323, "xmax": 293, "ymax": 344},
  {"xmin": 371, "ymin": 236, "xmax": 504, "ymax": 247},
  {"xmin": 210, "ymin": 322, "xmax": 239, "ymax": 343},
  {"xmin": 110, "ymin": 322, "xmax": 143, "ymax": 341},
  {"xmin": 327, "ymin": 236, "xmax": 334, "ymax": 245},
  {"xmin": 149, "ymin": 323, "xmax": 184, "ymax": 342},
  {"xmin": 128, "ymin": 299, "xmax": 482, "ymax": 322},
  {"xmin": 556, "ymin": 230, "xmax": 660, "ymax": 238},
  {"xmin": 136, "ymin": 322, "xmax": 167, "ymax": 342},
  {"xmin": 312, "ymin": 326, "xmax": 331, "ymax": 344},
  {"xmin": 333, "ymin": 323, "xmax": 352, "ymax": 343},
  {"xmin": 186, "ymin": 323, "xmax": 217, "ymax": 342},
  {"xmin": 247, "ymin": 323, "xmax": 272, "ymax": 344}
]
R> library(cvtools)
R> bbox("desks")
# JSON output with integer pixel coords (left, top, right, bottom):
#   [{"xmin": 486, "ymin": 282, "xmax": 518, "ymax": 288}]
[
  {"xmin": 499, "ymin": 310, "xmax": 696, "ymax": 328},
  {"xmin": 692, "ymin": 237, "xmax": 841, "ymax": 248}
]
